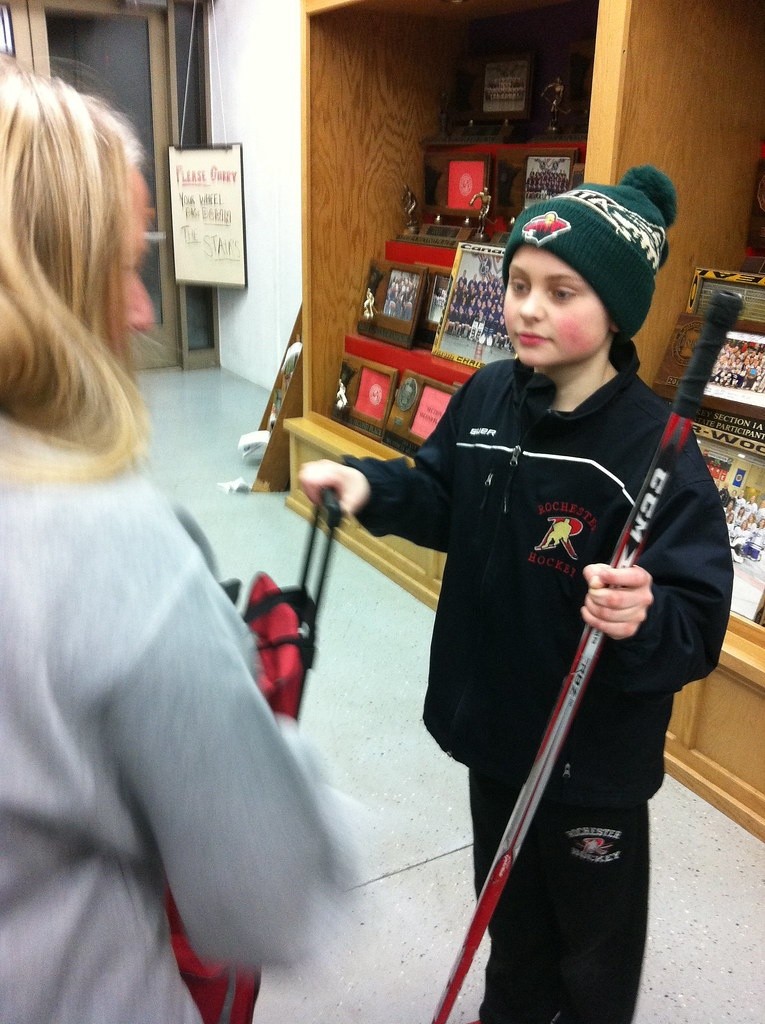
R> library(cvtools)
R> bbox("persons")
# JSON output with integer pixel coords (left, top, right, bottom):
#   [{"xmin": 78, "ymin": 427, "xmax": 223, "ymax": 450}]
[
  {"xmin": 384, "ymin": 275, "xmax": 416, "ymax": 319},
  {"xmin": 526, "ymin": 168, "xmax": 569, "ymax": 200},
  {"xmin": 433, "ymin": 288, "xmax": 447, "ymax": 307},
  {"xmin": 0, "ymin": 50, "xmax": 344, "ymax": 1024},
  {"xmin": 709, "ymin": 340, "xmax": 765, "ymax": 393},
  {"xmin": 445, "ymin": 269, "xmax": 515, "ymax": 354},
  {"xmin": 271, "ymin": 403, "xmax": 277, "ymax": 428},
  {"xmin": 300, "ymin": 165, "xmax": 735, "ymax": 1024},
  {"xmin": 703, "ymin": 450, "xmax": 765, "ymax": 563},
  {"xmin": 484, "ymin": 73, "xmax": 525, "ymax": 101}
]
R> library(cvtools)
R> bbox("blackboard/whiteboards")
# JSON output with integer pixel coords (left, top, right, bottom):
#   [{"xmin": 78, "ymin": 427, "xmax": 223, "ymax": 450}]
[{"xmin": 168, "ymin": 142, "xmax": 249, "ymax": 289}]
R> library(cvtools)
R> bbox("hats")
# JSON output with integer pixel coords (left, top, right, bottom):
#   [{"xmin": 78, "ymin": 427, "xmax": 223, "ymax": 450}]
[{"xmin": 504, "ymin": 165, "xmax": 678, "ymax": 345}]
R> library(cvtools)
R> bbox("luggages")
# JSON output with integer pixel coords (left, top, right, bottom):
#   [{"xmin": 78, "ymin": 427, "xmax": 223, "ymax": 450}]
[{"xmin": 166, "ymin": 486, "xmax": 343, "ymax": 1024}]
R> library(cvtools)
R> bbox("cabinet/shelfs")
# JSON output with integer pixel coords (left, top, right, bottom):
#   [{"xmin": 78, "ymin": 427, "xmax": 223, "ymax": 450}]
[{"xmin": 284, "ymin": 1, "xmax": 765, "ymax": 841}]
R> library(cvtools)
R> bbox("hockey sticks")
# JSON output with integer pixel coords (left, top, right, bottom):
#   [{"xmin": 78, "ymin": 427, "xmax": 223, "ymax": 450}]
[{"xmin": 429, "ymin": 285, "xmax": 745, "ymax": 1024}]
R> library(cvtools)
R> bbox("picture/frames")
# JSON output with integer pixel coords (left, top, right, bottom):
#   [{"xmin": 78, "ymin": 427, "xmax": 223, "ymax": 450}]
[
  {"xmin": 653, "ymin": 267, "xmax": 765, "ymax": 624},
  {"xmin": 330, "ymin": 146, "xmax": 584, "ymax": 461}
]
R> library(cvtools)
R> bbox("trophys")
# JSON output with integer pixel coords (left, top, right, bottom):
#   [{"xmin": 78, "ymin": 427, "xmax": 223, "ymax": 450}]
[
  {"xmin": 470, "ymin": 187, "xmax": 495, "ymax": 242},
  {"xmin": 402, "ymin": 185, "xmax": 419, "ymax": 235},
  {"xmin": 334, "ymin": 378, "xmax": 348, "ymax": 410},
  {"xmin": 361, "ymin": 289, "xmax": 377, "ymax": 320},
  {"xmin": 541, "ymin": 76, "xmax": 572, "ymax": 133}
]
